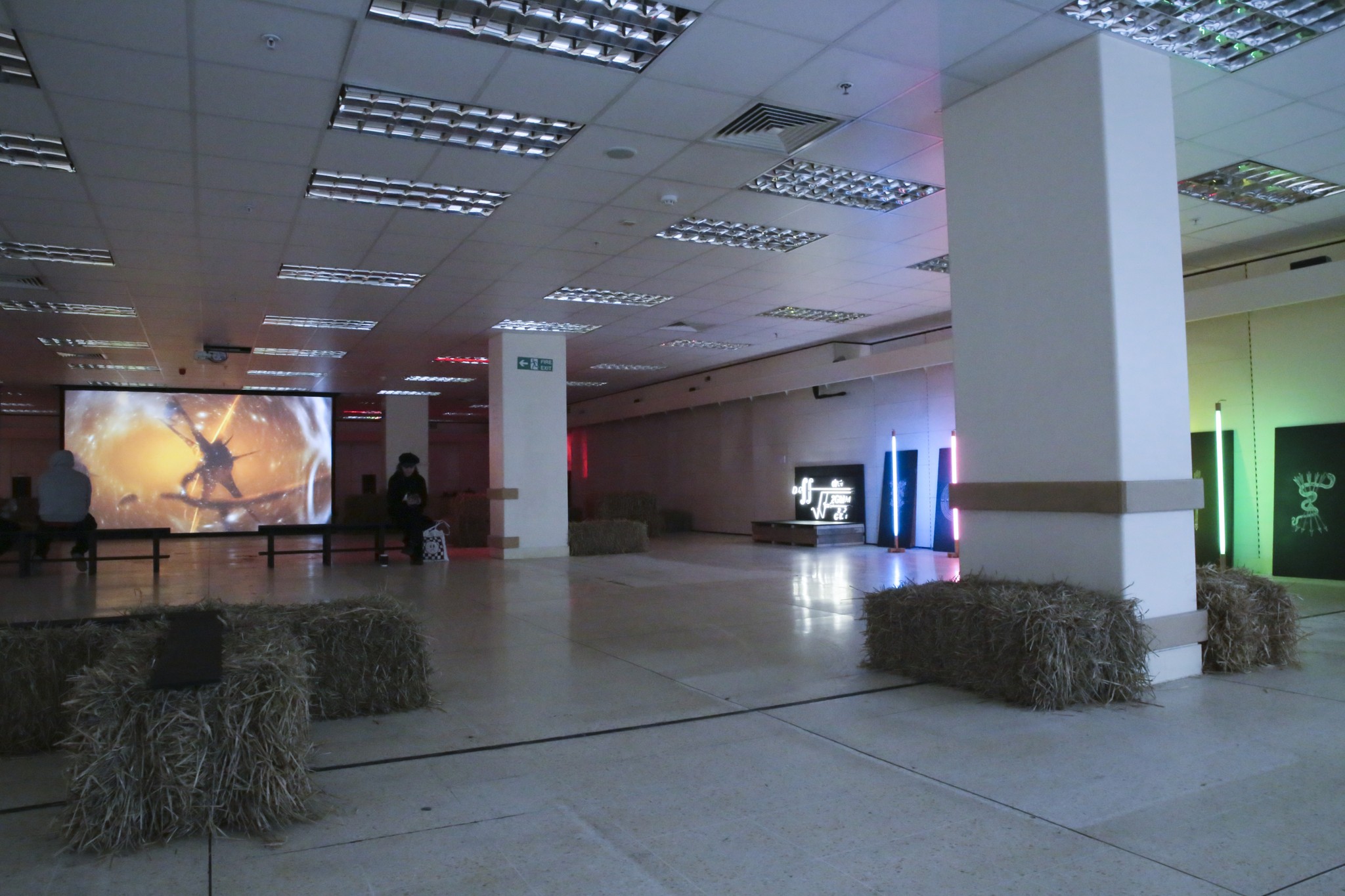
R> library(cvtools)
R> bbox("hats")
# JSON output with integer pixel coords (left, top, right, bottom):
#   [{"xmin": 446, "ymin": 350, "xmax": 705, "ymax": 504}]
[{"xmin": 398, "ymin": 453, "xmax": 420, "ymax": 467}]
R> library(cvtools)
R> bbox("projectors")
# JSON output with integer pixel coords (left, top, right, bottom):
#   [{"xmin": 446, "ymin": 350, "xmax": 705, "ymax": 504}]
[{"xmin": 192, "ymin": 351, "xmax": 211, "ymax": 360}]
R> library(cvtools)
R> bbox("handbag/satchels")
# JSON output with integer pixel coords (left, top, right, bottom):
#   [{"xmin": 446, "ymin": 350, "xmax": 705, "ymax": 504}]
[{"xmin": 423, "ymin": 520, "xmax": 451, "ymax": 563}]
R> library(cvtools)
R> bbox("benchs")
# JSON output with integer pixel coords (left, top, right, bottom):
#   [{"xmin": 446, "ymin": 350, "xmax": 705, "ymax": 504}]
[
  {"xmin": 258, "ymin": 523, "xmax": 436, "ymax": 568},
  {"xmin": 1, "ymin": 528, "xmax": 169, "ymax": 574}
]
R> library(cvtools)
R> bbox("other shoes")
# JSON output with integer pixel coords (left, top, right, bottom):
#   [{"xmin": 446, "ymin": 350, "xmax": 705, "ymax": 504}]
[
  {"xmin": 72, "ymin": 549, "xmax": 87, "ymax": 572},
  {"xmin": 400, "ymin": 547, "xmax": 424, "ymax": 565},
  {"xmin": 31, "ymin": 554, "xmax": 43, "ymax": 576}
]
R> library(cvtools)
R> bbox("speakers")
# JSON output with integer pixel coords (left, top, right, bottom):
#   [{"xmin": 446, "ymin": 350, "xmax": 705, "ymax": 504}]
[
  {"xmin": 11, "ymin": 473, "xmax": 33, "ymax": 503},
  {"xmin": 361, "ymin": 473, "xmax": 376, "ymax": 495}
]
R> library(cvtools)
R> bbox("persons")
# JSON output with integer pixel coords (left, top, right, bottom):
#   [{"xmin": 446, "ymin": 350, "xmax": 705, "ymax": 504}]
[
  {"xmin": 36, "ymin": 449, "xmax": 97, "ymax": 574},
  {"xmin": 387, "ymin": 451, "xmax": 432, "ymax": 565}
]
